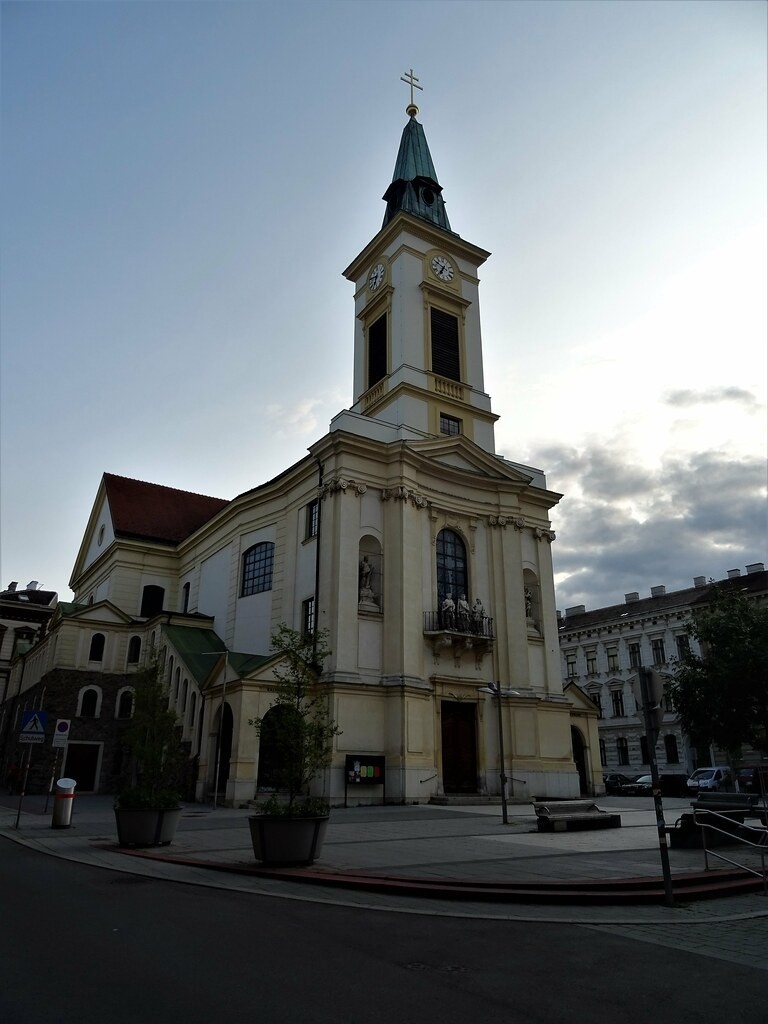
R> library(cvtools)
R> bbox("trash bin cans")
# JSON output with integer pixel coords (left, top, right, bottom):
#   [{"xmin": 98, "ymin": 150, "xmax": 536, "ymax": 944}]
[{"xmin": 51, "ymin": 778, "xmax": 76, "ymax": 830}]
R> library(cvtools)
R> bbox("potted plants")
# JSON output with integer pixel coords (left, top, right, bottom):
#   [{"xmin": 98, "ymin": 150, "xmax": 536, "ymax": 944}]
[
  {"xmin": 104, "ymin": 640, "xmax": 192, "ymax": 846},
  {"xmin": 248, "ymin": 621, "xmax": 345, "ymax": 861}
]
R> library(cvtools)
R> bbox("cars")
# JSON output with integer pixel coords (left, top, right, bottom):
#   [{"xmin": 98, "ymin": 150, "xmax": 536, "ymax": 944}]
[
  {"xmin": 621, "ymin": 773, "xmax": 688, "ymax": 797},
  {"xmin": 602, "ymin": 772, "xmax": 630, "ymax": 796}
]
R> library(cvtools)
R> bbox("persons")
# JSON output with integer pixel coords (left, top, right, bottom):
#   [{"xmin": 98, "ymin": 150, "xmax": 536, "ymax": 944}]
[
  {"xmin": 524, "ymin": 587, "xmax": 534, "ymax": 620},
  {"xmin": 359, "ymin": 554, "xmax": 375, "ymax": 603},
  {"xmin": 444, "ymin": 593, "xmax": 489, "ymax": 633}
]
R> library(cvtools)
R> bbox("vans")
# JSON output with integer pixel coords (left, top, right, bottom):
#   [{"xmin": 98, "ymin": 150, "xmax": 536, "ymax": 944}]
[
  {"xmin": 686, "ymin": 765, "xmax": 731, "ymax": 792},
  {"xmin": 733, "ymin": 762, "xmax": 768, "ymax": 794}
]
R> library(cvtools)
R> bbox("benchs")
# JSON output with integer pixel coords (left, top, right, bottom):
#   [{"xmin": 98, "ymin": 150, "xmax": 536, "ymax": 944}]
[
  {"xmin": 532, "ymin": 800, "xmax": 621, "ymax": 832},
  {"xmin": 666, "ymin": 809, "xmax": 750, "ymax": 849},
  {"xmin": 690, "ymin": 791, "xmax": 760, "ymax": 818}
]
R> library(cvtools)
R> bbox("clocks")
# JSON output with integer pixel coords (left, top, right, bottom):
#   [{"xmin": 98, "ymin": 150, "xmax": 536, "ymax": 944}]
[
  {"xmin": 369, "ymin": 264, "xmax": 385, "ymax": 289},
  {"xmin": 432, "ymin": 255, "xmax": 454, "ymax": 280}
]
